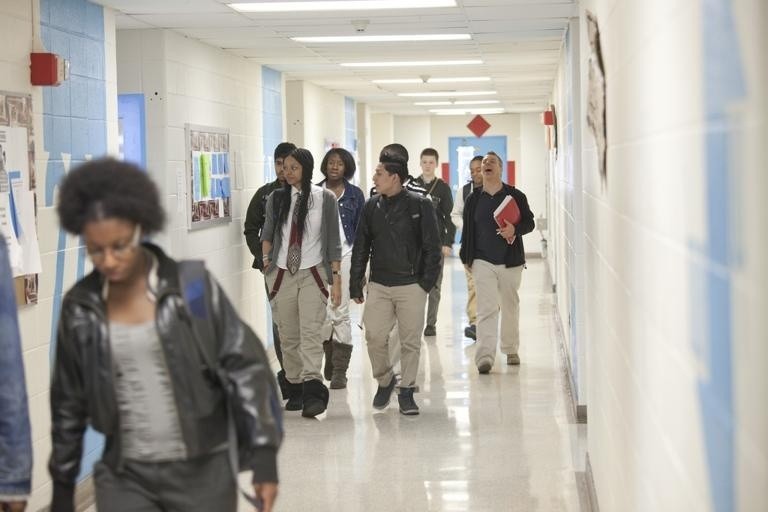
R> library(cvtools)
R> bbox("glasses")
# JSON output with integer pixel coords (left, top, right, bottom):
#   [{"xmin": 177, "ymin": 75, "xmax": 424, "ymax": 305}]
[{"xmin": 83, "ymin": 224, "xmax": 144, "ymax": 262}]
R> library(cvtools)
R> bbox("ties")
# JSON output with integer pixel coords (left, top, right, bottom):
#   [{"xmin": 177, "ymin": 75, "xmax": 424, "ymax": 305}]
[{"xmin": 285, "ymin": 190, "xmax": 304, "ymax": 277}]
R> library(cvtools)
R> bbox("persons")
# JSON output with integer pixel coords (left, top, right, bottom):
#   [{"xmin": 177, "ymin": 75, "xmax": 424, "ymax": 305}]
[
  {"xmin": 259, "ymin": 148, "xmax": 342, "ymax": 419},
  {"xmin": 451, "ymin": 154, "xmax": 484, "ymax": 341},
  {"xmin": 244, "ymin": 143, "xmax": 305, "ymax": 403},
  {"xmin": 415, "ymin": 148, "xmax": 455, "ymax": 338},
  {"xmin": 312, "ymin": 145, "xmax": 366, "ymax": 392},
  {"xmin": 0, "ymin": 235, "xmax": 33, "ymax": 512},
  {"xmin": 459, "ymin": 151, "xmax": 535, "ymax": 375},
  {"xmin": 367, "ymin": 142, "xmax": 433, "ymax": 201},
  {"xmin": 46, "ymin": 158, "xmax": 284, "ymax": 512},
  {"xmin": 347, "ymin": 155, "xmax": 443, "ymax": 415}
]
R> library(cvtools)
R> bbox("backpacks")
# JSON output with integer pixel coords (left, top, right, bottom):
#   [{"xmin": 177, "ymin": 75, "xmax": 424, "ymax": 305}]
[
  {"xmin": 446, "ymin": 215, "xmax": 458, "ymax": 249},
  {"xmin": 175, "ymin": 257, "xmax": 286, "ymax": 476}
]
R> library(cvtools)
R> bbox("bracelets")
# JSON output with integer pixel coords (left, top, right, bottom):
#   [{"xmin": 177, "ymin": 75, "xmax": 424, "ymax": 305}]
[
  {"xmin": 262, "ymin": 254, "xmax": 269, "ymax": 260},
  {"xmin": 332, "ymin": 270, "xmax": 341, "ymax": 274}
]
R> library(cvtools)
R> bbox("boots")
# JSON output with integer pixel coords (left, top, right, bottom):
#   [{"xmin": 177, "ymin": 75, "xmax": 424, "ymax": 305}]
[
  {"xmin": 322, "ymin": 338, "xmax": 354, "ymax": 391},
  {"xmin": 284, "ymin": 377, "xmax": 306, "ymax": 412},
  {"xmin": 301, "ymin": 377, "xmax": 332, "ymax": 419}
]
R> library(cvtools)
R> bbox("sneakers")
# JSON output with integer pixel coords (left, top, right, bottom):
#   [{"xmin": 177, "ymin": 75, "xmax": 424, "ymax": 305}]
[
  {"xmin": 395, "ymin": 387, "xmax": 421, "ymax": 416},
  {"xmin": 476, "ymin": 355, "xmax": 495, "ymax": 375},
  {"xmin": 505, "ymin": 351, "xmax": 521, "ymax": 366},
  {"xmin": 371, "ymin": 373, "xmax": 398, "ymax": 411},
  {"xmin": 464, "ymin": 323, "xmax": 478, "ymax": 342},
  {"xmin": 423, "ymin": 323, "xmax": 436, "ymax": 337}
]
